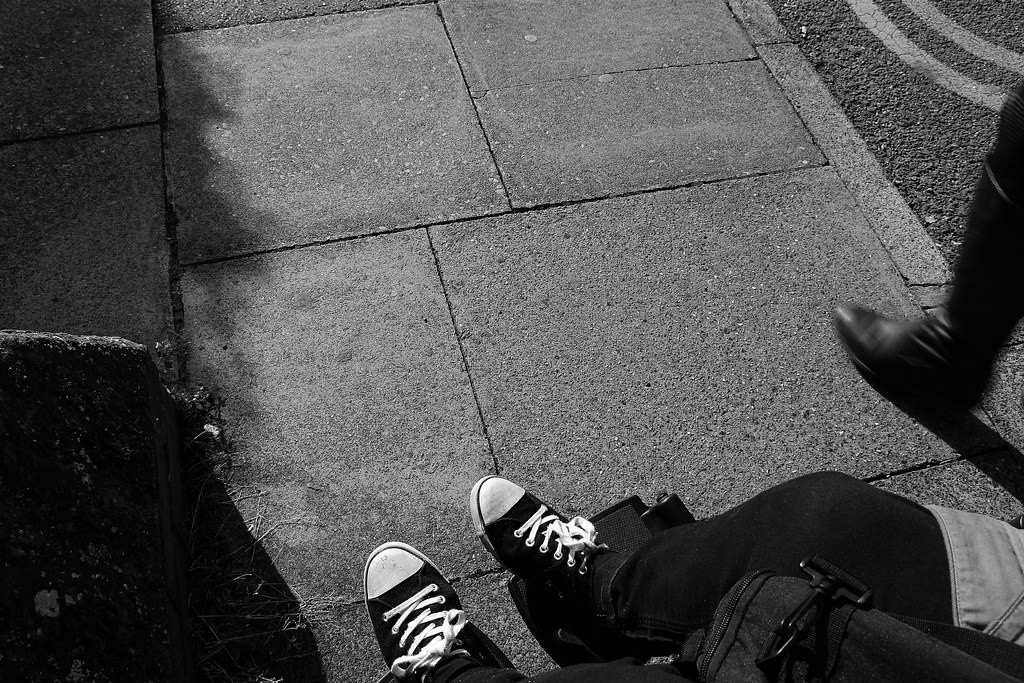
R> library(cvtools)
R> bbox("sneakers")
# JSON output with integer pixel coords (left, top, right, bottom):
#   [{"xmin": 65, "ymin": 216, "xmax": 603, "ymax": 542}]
[
  {"xmin": 470, "ymin": 476, "xmax": 607, "ymax": 593},
  {"xmin": 362, "ymin": 542, "xmax": 475, "ymax": 671}
]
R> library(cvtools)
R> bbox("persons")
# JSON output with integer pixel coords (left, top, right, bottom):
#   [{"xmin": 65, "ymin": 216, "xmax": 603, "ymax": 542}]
[
  {"xmin": 831, "ymin": 76, "xmax": 1024, "ymax": 432},
  {"xmin": 363, "ymin": 474, "xmax": 1024, "ymax": 683}
]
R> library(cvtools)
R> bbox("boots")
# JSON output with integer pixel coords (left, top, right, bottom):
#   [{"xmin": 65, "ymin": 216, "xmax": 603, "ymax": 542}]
[{"xmin": 833, "ymin": 156, "xmax": 1024, "ymax": 403}]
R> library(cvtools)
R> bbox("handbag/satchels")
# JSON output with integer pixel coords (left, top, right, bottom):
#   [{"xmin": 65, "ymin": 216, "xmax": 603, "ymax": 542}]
[{"xmin": 667, "ymin": 558, "xmax": 1024, "ymax": 683}]
